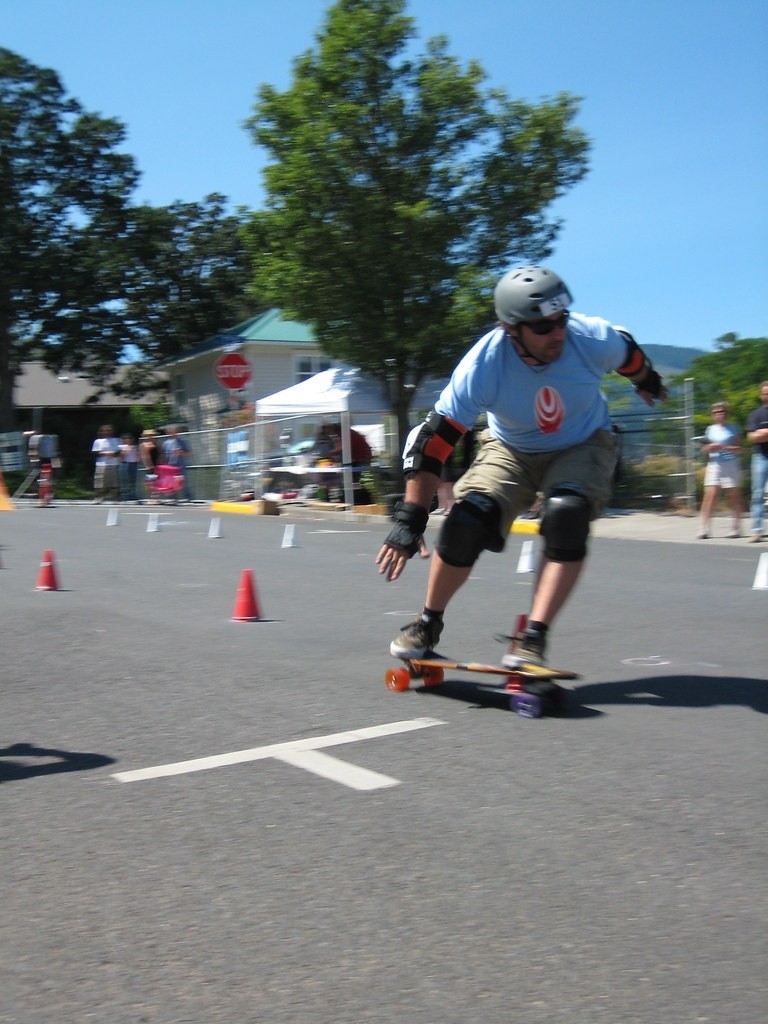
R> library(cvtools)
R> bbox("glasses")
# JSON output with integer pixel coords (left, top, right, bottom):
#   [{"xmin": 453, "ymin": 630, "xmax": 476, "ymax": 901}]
[
  {"xmin": 712, "ymin": 410, "xmax": 723, "ymax": 414},
  {"xmin": 521, "ymin": 309, "xmax": 570, "ymax": 335}
]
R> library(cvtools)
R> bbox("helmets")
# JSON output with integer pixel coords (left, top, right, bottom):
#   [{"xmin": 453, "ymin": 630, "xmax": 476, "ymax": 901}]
[{"xmin": 494, "ymin": 266, "xmax": 572, "ymax": 324}]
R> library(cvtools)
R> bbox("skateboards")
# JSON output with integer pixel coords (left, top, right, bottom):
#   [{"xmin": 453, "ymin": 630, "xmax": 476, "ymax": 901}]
[{"xmin": 384, "ymin": 647, "xmax": 584, "ymax": 720}]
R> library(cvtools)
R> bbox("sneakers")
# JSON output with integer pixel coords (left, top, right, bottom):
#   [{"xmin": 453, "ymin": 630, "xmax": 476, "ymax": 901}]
[
  {"xmin": 390, "ymin": 615, "xmax": 444, "ymax": 658},
  {"xmin": 493, "ymin": 630, "xmax": 548, "ymax": 668}
]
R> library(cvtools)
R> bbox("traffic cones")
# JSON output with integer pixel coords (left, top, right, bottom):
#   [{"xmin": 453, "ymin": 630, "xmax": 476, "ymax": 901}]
[
  {"xmin": 507, "ymin": 611, "xmax": 530, "ymax": 691},
  {"xmin": 36, "ymin": 549, "xmax": 62, "ymax": 592},
  {"xmin": 229, "ymin": 568, "xmax": 262, "ymax": 623}
]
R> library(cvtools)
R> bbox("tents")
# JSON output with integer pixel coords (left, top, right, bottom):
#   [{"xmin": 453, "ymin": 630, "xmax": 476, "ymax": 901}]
[{"xmin": 252, "ymin": 359, "xmax": 452, "ymax": 509}]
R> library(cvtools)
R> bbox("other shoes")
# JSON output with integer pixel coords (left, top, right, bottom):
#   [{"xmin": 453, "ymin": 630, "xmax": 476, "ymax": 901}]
[
  {"xmin": 697, "ymin": 529, "xmax": 708, "ymax": 537},
  {"xmin": 750, "ymin": 533, "xmax": 763, "ymax": 542},
  {"xmin": 728, "ymin": 528, "xmax": 742, "ymax": 537}
]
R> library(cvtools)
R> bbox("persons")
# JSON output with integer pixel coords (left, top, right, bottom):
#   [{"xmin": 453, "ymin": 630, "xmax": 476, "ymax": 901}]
[
  {"xmin": 372, "ymin": 267, "xmax": 669, "ymax": 668},
  {"xmin": 30, "ymin": 384, "xmax": 768, "ymax": 543}
]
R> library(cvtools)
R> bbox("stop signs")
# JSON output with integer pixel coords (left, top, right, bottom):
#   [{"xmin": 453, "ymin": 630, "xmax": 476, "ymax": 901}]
[{"xmin": 216, "ymin": 351, "xmax": 251, "ymax": 390}]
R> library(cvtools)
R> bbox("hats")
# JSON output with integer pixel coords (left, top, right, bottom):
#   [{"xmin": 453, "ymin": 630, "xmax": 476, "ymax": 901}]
[{"xmin": 142, "ymin": 429, "xmax": 158, "ymax": 436}]
